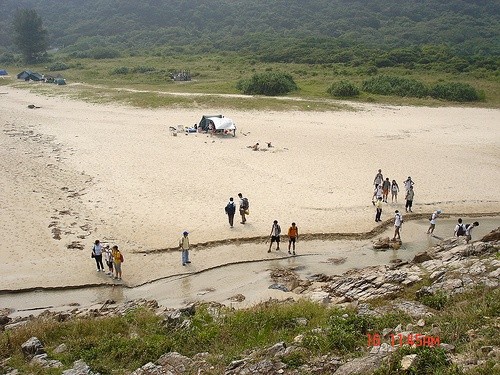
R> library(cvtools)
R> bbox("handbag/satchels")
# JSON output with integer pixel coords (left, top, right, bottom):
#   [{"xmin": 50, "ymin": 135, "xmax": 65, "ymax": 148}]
[
  {"xmin": 91, "ymin": 249, "xmax": 96, "ymax": 258},
  {"xmin": 245, "ymin": 208, "xmax": 250, "ymax": 215}
]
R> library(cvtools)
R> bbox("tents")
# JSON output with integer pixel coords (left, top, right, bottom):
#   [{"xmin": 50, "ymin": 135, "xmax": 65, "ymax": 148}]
[
  {"xmin": 17, "ymin": 70, "xmax": 42, "ymax": 82},
  {"xmin": 0, "ymin": 70, "xmax": 8, "ymax": 75},
  {"xmin": 46, "ymin": 76, "xmax": 66, "ymax": 85},
  {"xmin": 199, "ymin": 114, "xmax": 236, "ymax": 138}
]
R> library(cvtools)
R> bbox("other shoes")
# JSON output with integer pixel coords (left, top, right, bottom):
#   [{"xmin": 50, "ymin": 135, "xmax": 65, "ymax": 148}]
[
  {"xmin": 288, "ymin": 250, "xmax": 291, "ymax": 254},
  {"xmin": 106, "ymin": 271, "xmax": 111, "ymax": 275},
  {"xmin": 118, "ymin": 277, "xmax": 122, "ymax": 281},
  {"xmin": 113, "ymin": 276, "xmax": 118, "ymax": 280},
  {"xmin": 267, "ymin": 248, "xmax": 272, "ymax": 253},
  {"xmin": 375, "ymin": 218, "xmax": 381, "ymax": 222},
  {"xmin": 293, "ymin": 251, "xmax": 296, "ymax": 255},
  {"xmin": 103, "ymin": 268, "xmax": 105, "ymax": 272},
  {"xmin": 109, "ymin": 273, "xmax": 114, "ymax": 278},
  {"xmin": 275, "ymin": 246, "xmax": 279, "ymax": 250},
  {"xmin": 97, "ymin": 268, "xmax": 100, "ymax": 272},
  {"xmin": 183, "ymin": 260, "xmax": 191, "ymax": 266}
]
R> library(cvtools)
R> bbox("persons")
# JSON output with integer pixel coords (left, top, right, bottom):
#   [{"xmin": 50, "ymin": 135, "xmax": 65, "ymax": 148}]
[
  {"xmin": 224, "ymin": 197, "xmax": 236, "ymax": 228},
  {"xmin": 393, "ymin": 210, "xmax": 404, "ymax": 240},
  {"xmin": 371, "ymin": 169, "xmax": 415, "ymax": 222},
  {"xmin": 426, "ymin": 210, "xmax": 441, "ymax": 234},
  {"xmin": 265, "ymin": 220, "xmax": 282, "ymax": 253},
  {"xmin": 41, "ymin": 73, "xmax": 44, "ymax": 82},
  {"xmin": 286, "ymin": 222, "xmax": 298, "ymax": 255},
  {"xmin": 92, "ymin": 240, "xmax": 122, "ymax": 281},
  {"xmin": 466, "ymin": 221, "xmax": 480, "ymax": 240},
  {"xmin": 237, "ymin": 193, "xmax": 249, "ymax": 224},
  {"xmin": 454, "ymin": 217, "xmax": 467, "ymax": 237},
  {"xmin": 181, "ymin": 231, "xmax": 192, "ymax": 266}
]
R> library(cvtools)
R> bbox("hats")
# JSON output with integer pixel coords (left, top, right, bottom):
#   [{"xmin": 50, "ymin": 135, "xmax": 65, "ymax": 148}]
[
  {"xmin": 103, "ymin": 243, "xmax": 110, "ymax": 248},
  {"xmin": 274, "ymin": 220, "xmax": 278, "ymax": 223},
  {"xmin": 94, "ymin": 240, "xmax": 101, "ymax": 244},
  {"xmin": 184, "ymin": 231, "xmax": 189, "ymax": 236},
  {"xmin": 113, "ymin": 246, "xmax": 118, "ymax": 249},
  {"xmin": 437, "ymin": 209, "xmax": 442, "ymax": 214},
  {"xmin": 292, "ymin": 222, "xmax": 296, "ymax": 225}
]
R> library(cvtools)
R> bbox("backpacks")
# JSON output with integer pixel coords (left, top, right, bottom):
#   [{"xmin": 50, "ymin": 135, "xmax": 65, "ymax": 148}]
[
  {"xmin": 119, "ymin": 254, "xmax": 124, "ymax": 262},
  {"xmin": 429, "ymin": 213, "xmax": 437, "ymax": 220},
  {"xmin": 241, "ymin": 198, "xmax": 249, "ymax": 210},
  {"xmin": 456, "ymin": 224, "xmax": 464, "ymax": 236},
  {"xmin": 227, "ymin": 202, "xmax": 234, "ymax": 214},
  {"xmin": 465, "ymin": 223, "xmax": 473, "ymax": 229}
]
